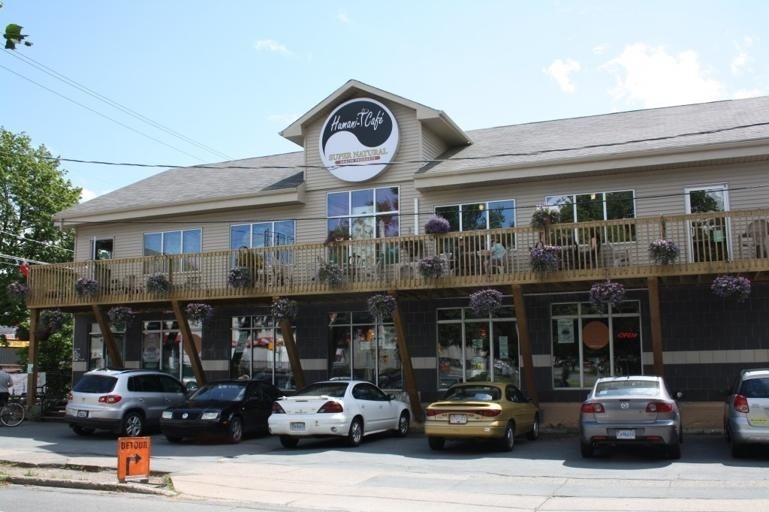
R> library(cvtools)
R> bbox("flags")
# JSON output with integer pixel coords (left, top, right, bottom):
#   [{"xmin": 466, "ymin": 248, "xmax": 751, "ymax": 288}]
[{"xmin": 20, "ymin": 259, "xmax": 32, "ymax": 280}]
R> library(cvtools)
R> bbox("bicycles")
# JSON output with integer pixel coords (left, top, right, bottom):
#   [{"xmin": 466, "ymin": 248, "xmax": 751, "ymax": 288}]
[{"xmin": 0, "ymin": 393, "xmax": 25, "ymax": 427}]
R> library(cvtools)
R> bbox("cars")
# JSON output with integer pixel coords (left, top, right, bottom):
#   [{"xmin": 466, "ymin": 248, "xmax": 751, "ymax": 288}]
[
  {"xmin": 267, "ymin": 379, "xmax": 411, "ymax": 447},
  {"xmin": 424, "ymin": 383, "xmax": 540, "ymax": 451},
  {"xmin": 160, "ymin": 380, "xmax": 287, "ymax": 445},
  {"xmin": 578, "ymin": 375, "xmax": 682, "ymax": 459},
  {"xmin": 724, "ymin": 369, "xmax": 769, "ymax": 458}
]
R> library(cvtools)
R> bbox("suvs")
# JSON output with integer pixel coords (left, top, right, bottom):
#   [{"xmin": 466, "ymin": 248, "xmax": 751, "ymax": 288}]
[{"xmin": 65, "ymin": 368, "xmax": 191, "ymax": 439}]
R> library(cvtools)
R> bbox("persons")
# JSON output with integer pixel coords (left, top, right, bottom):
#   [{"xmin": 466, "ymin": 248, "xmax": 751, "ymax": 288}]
[
  {"xmin": 0, "ymin": 365, "xmax": 15, "ymax": 429},
  {"xmin": 554, "ymin": 352, "xmax": 640, "ymax": 388}
]
